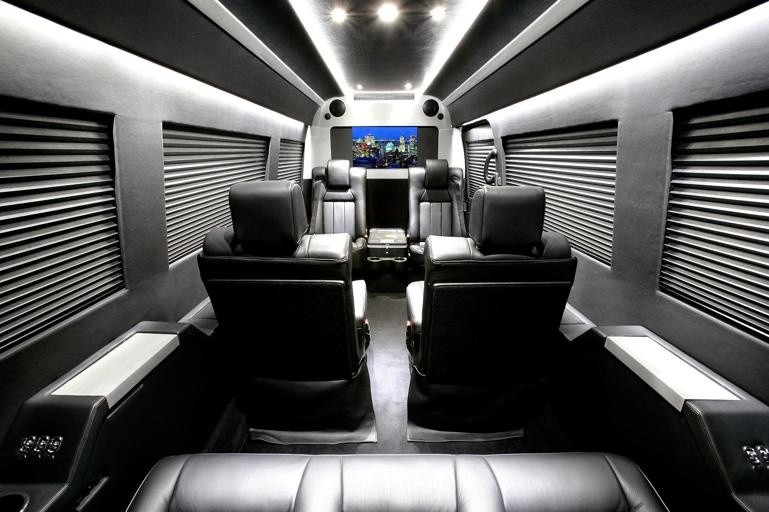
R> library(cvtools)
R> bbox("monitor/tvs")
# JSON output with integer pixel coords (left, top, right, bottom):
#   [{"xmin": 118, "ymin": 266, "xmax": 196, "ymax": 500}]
[{"xmin": 352, "ymin": 126, "xmax": 418, "ymax": 168}]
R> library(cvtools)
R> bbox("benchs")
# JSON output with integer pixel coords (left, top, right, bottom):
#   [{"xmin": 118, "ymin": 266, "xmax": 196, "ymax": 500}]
[{"xmin": 125, "ymin": 449, "xmax": 670, "ymax": 511}]
